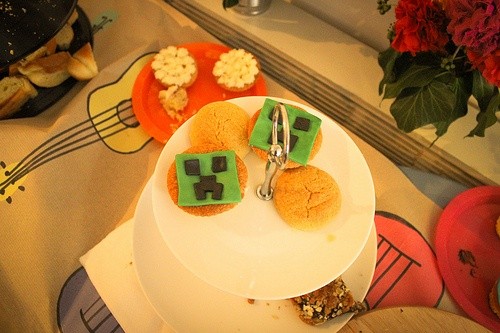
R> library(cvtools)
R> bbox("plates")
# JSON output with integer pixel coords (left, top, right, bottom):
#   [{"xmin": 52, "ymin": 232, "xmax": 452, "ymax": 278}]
[
  {"xmin": 152, "ymin": 96, "xmax": 375, "ymax": 300},
  {"xmin": 0, "ymin": 0, "xmax": 95, "ymax": 122},
  {"xmin": 436, "ymin": 185, "xmax": 500, "ymax": 333},
  {"xmin": 132, "ymin": 43, "xmax": 267, "ymax": 144},
  {"xmin": 131, "ymin": 171, "xmax": 377, "ymax": 333}
]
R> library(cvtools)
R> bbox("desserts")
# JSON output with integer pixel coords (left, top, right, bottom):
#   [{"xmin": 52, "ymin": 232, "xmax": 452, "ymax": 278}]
[
  {"xmin": 151, "ymin": 46, "xmax": 198, "ymax": 91},
  {"xmin": 289, "ymin": 273, "xmax": 367, "ymax": 327},
  {"xmin": 489, "ymin": 277, "xmax": 500, "ymax": 319},
  {"xmin": 212, "ymin": 48, "xmax": 260, "ymax": 92}
]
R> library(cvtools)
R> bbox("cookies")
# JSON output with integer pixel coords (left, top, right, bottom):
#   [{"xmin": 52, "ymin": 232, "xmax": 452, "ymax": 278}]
[{"xmin": 166, "ymin": 102, "xmax": 342, "ymax": 232}]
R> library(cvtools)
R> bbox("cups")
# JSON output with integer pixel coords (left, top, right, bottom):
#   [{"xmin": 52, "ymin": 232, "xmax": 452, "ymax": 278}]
[{"xmin": 223, "ymin": 0, "xmax": 274, "ymax": 14}]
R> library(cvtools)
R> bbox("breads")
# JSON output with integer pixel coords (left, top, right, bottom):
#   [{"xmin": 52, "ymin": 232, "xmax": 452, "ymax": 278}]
[{"xmin": 0, "ymin": 7, "xmax": 100, "ymax": 120}]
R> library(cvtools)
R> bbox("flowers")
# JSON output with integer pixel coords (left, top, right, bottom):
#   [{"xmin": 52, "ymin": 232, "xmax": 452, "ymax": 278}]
[{"xmin": 375, "ymin": 0, "xmax": 500, "ymax": 144}]
